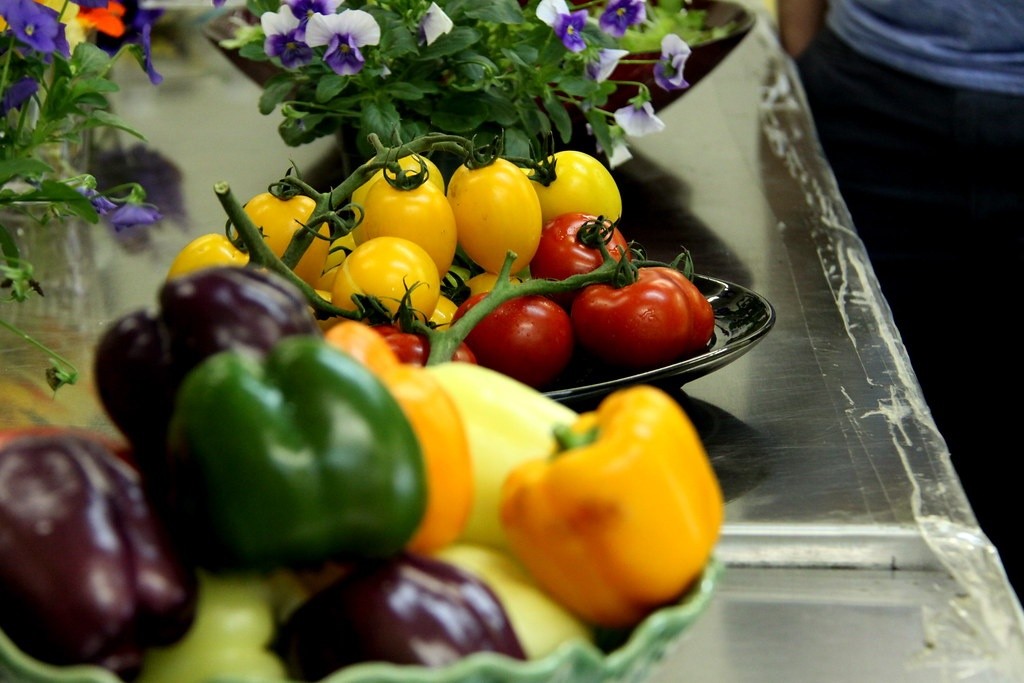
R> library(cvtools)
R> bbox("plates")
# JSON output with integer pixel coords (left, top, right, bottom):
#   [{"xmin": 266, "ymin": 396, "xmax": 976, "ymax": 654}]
[
  {"xmin": 529, "ymin": 260, "xmax": 775, "ymax": 412},
  {"xmin": 0, "ymin": 554, "xmax": 722, "ymax": 683}
]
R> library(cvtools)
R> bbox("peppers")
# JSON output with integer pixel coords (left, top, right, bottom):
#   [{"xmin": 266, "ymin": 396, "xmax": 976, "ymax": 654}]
[{"xmin": 0, "ymin": 265, "xmax": 725, "ymax": 683}]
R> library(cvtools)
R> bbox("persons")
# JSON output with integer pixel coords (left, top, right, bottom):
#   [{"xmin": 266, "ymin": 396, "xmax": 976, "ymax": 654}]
[{"xmin": 779, "ymin": 0, "xmax": 1023, "ymax": 604}]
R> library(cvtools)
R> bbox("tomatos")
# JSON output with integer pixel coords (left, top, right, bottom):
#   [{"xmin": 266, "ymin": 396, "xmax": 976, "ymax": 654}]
[{"xmin": 155, "ymin": 133, "xmax": 714, "ymax": 384}]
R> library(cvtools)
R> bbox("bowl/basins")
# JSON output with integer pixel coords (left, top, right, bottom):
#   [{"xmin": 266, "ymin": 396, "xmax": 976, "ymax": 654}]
[{"xmin": 204, "ymin": 0, "xmax": 761, "ymax": 153}]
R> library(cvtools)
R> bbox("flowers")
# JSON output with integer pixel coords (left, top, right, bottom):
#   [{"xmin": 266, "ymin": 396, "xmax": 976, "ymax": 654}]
[
  {"xmin": 228, "ymin": 0, "xmax": 693, "ymax": 173},
  {"xmin": 0, "ymin": 0, "xmax": 164, "ymax": 389}
]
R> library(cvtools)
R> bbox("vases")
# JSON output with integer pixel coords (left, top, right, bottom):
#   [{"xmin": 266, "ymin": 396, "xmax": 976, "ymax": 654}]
[{"xmin": 0, "ymin": 107, "xmax": 110, "ymax": 348}]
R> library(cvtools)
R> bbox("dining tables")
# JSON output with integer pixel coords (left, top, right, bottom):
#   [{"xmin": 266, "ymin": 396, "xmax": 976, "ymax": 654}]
[{"xmin": 0, "ymin": 0, "xmax": 1024, "ymax": 683}]
